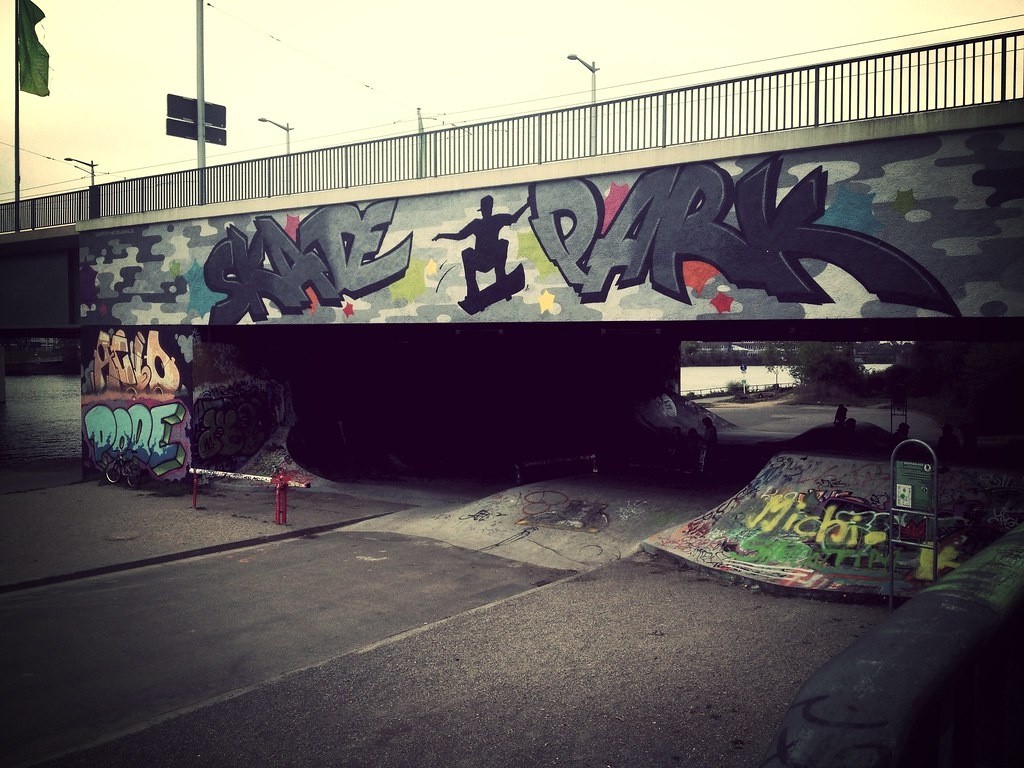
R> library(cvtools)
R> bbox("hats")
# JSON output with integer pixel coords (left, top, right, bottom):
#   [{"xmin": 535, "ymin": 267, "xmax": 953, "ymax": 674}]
[
  {"xmin": 899, "ymin": 423, "xmax": 911, "ymax": 430},
  {"xmin": 940, "ymin": 424, "xmax": 953, "ymax": 432}
]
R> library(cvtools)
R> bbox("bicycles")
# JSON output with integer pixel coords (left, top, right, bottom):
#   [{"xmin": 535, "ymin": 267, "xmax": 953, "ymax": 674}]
[{"xmin": 104, "ymin": 446, "xmax": 142, "ymax": 487}]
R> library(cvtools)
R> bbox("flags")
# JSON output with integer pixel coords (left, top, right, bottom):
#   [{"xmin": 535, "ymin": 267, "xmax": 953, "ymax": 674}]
[{"xmin": 16, "ymin": 0, "xmax": 50, "ymax": 97}]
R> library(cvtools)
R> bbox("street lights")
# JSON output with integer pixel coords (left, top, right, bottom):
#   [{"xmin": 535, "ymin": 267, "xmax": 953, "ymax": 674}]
[
  {"xmin": 64, "ymin": 158, "xmax": 98, "ymax": 186},
  {"xmin": 258, "ymin": 117, "xmax": 294, "ymax": 195},
  {"xmin": 567, "ymin": 53, "xmax": 599, "ymax": 154}
]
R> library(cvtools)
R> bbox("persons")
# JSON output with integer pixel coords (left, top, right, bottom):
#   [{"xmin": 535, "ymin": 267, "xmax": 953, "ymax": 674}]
[
  {"xmin": 935, "ymin": 424, "xmax": 959, "ymax": 457},
  {"xmin": 833, "ymin": 403, "xmax": 861, "ymax": 452},
  {"xmin": 886, "ymin": 422, "xmax": 914, "ymax": 461},
  {"xmin": 667, "ymin": 416, "xmax": 720, "ymax": 479}
]
